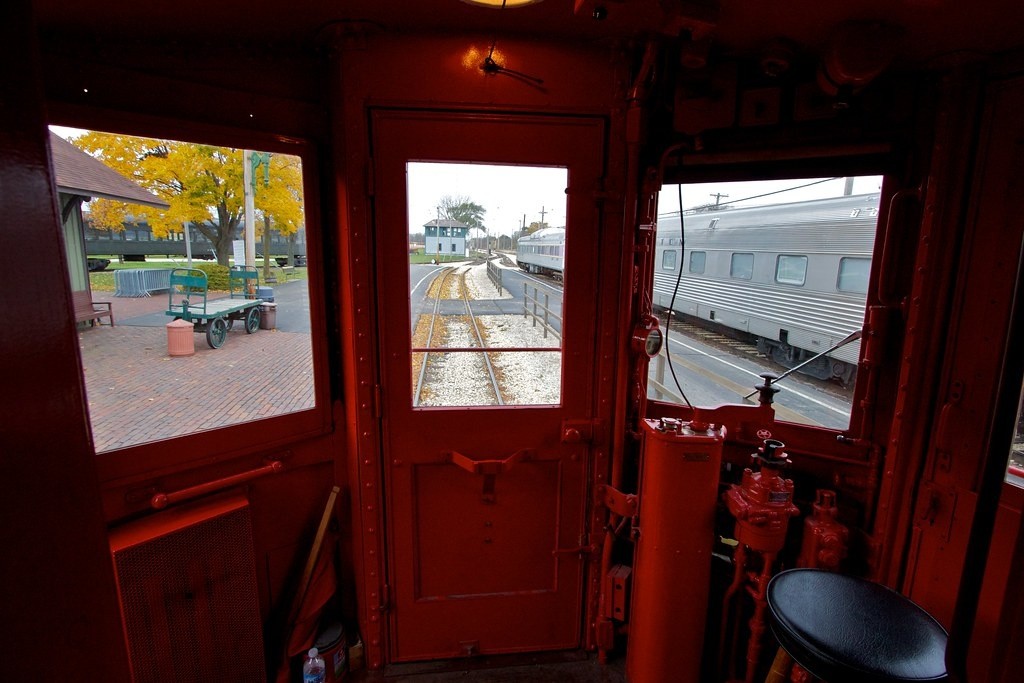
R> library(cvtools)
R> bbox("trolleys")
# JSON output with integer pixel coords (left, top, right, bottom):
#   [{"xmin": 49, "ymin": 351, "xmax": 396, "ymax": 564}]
[{"xmin": 165, "ymin": 265, "xmax": 263, "ymax": 349}]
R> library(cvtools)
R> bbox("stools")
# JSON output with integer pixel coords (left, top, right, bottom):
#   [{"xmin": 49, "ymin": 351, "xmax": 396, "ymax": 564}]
[{"xmin": 761, "ymin": 566, "xmax": 948, "ymax": 683}]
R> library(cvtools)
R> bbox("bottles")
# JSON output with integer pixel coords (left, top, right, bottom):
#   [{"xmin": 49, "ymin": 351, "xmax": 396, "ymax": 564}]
[{"xmin": 304, "ymin": 648, "xmax": 326, "ymax": 683}]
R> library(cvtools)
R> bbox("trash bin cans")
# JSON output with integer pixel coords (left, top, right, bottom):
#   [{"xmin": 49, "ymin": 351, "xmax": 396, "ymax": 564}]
[
  {"xmin": 254, "ymin": 287, "xmax": 274, "ymax": 303},
  {"xmin": 260, "ymin": 302, "xmax": 278, "ymax": 330}
]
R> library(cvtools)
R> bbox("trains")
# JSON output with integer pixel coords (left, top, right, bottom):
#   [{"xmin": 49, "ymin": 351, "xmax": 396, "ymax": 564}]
[
  {"xmin": 79, "ymin": 211, "xmax": 308, "ymax": 267},
  {"xmin": 515, "ymin": 191, "xmax": 879, "ymax": 387}
]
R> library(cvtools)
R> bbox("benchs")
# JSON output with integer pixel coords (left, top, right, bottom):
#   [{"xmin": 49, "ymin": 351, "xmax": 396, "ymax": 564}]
[{"xmin": 73, "ymin": 288, "xmax": 114, "ymax": 329}]
